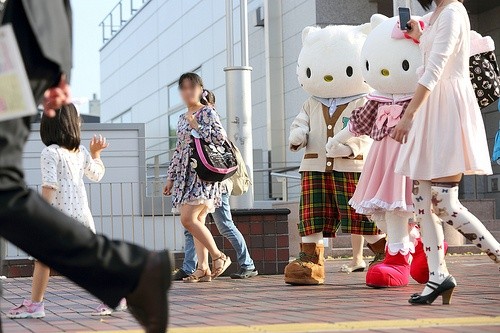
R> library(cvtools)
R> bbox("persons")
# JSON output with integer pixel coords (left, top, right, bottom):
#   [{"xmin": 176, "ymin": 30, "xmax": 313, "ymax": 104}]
[
  {"xmin": 338, "ymin": 232, "xmax": 366, "ymax": 272},
  {"xmin": 0, "ymin": 0, "xmax": 175, "ymax": 333},
  {"xmin": 5, "ymin": 103, "xmax": 127, "ymax": 319},
  {"xmin": 390, "ymin": 0, "xmax": 500, "ymax": 306},
  {"xmin": 164, "ymin": 72, "xmax": 258, "ymax": 283}
]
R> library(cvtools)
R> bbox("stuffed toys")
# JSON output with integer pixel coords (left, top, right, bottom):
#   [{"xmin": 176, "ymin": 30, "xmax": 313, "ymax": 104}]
[{"xmin": 285, "ymin": 14, "xmax": 449, "ymax": 286}]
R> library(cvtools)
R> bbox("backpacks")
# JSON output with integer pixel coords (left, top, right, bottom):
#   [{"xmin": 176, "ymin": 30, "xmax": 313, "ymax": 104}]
[{"xmin": 224, "ymin": 137, "xmax": 252, "ymax": 196}]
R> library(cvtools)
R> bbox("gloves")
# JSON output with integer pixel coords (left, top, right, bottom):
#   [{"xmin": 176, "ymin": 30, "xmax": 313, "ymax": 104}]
[
  {"xmin": 326, "ymin": 129, "xmax": 352, "ymax": 158},
  {"xmin": 288, "ymin": 126, "xmax": 306, "ymax": 146}
]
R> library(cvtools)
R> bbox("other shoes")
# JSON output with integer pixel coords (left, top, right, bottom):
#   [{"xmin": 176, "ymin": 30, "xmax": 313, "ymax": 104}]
[{"xmin": 338, "ymin": 260, "xmax": 366, "ymax": 272}]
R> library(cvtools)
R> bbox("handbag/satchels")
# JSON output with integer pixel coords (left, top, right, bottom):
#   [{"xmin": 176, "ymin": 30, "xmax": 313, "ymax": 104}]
[
  {"xmin": 191, "ymin": 107, "xmax": 238, "ymax": 181},
  {"xmin": 468, "ymin": 35, "xmax": 500, "ymax": 110}
]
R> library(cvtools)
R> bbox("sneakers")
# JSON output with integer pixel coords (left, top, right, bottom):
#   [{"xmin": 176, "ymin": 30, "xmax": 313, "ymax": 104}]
[
  {"xmin": 91, "ymin": 298, "xmax": 128, "ymax": 316},
  {"xmin": 231, "ymin": 263, "xmax": 258, "ymax": 278},
  {"xmin": 7, "ymin": 299, "xmax": 45, "ymax": 318},
  {"xmin": 172, "ymin": 269, "xmax": 187, "ymax": 280}
]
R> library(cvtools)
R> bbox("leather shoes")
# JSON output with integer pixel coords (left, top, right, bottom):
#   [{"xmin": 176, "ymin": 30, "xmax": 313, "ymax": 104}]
[{"xmin": 125, "ymin": 251, "xmax": 171, "ymax": 333}]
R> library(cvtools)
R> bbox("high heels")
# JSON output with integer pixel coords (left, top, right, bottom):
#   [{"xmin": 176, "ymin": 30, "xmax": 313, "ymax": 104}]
[
  {"xmin": 182, "ymin": 266, "xmax": 211, "ymax": 283},
  {"xmin": 408, "ymin": 274, "xmax": 457, "ymax": 304},
  {"xmin": 211, "ymin": 252, "xmax": 231, "ymax": 279}
]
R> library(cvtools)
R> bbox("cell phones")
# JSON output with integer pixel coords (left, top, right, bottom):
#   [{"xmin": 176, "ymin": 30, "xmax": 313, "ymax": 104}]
[{"xmin": 399, "ymin": 7, "xmax": 411, "ymax": 30}]
